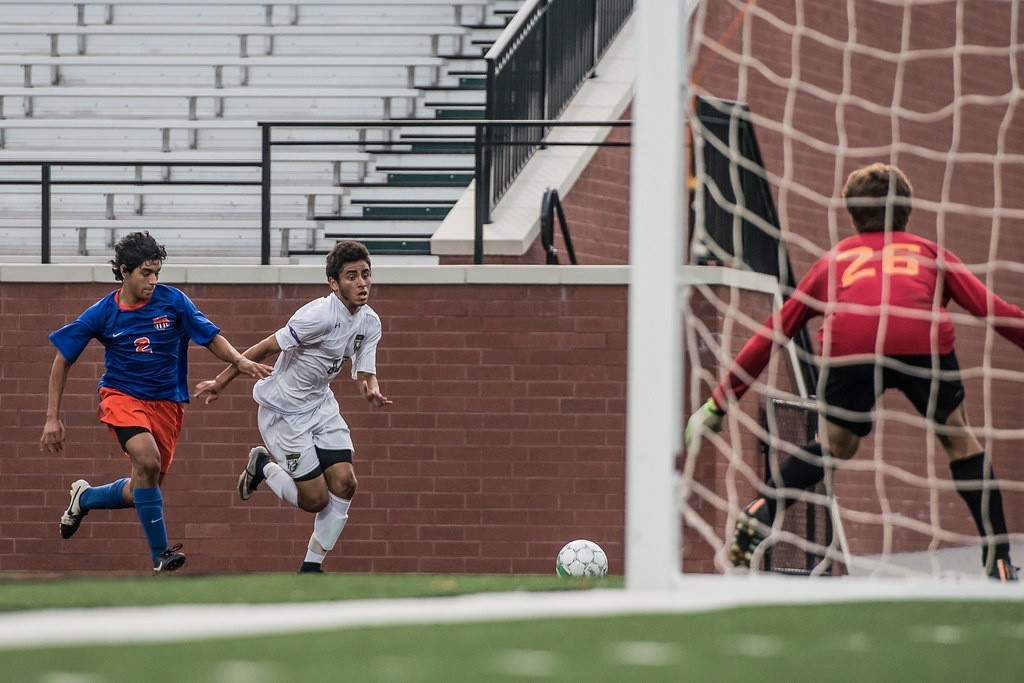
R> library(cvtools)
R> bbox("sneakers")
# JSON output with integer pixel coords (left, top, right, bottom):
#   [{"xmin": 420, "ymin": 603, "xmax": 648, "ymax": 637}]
[
  {"xmin": 237, "ymin": 445, "xmax": 273, "ymax": 502},
  {"xmin": 730, "ymin": 498, "xmax": 773, "ymax": 572},
  {"xmin": 989, "ymin": 559, "xmax": 1018, "ymax": 580},
  {"xmin": 153, "ymin": 550, "xmax": 186, "ymax": 574},
  {"xmin": 59, "ymin": 479, "xmax": 92, "ymax": 540}
]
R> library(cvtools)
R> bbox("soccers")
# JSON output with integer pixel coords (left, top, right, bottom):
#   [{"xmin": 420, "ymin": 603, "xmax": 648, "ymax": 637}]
[{"xmin": 555, "ymin": 539, "xmax": 608, "ymax": 579}]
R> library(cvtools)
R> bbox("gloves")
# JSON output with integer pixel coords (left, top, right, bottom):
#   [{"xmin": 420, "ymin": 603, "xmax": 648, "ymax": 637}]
[{"xmin": 683, "ymin": 396, "xmax": 724, "ymax": 447}]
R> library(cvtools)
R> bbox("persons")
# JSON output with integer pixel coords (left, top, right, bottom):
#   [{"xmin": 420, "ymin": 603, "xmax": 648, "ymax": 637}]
[
  {"xmin": 194, "ymin": 242, "xmax": 394, "ymax": 573},
  {"xmin": 685, "ymin": 163, "xmax": 1023, "ymax": 583},
  {"xmin": 39, "ymin": 230, "xmax": 277, "ymax": 573}
]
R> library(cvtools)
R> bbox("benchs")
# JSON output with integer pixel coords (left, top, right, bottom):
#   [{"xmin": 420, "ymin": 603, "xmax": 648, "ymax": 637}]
[
  {"xmin": 0, "ymin": 88, "xmax": 433, "ymax": 121},
  {"xmin": 1, "ymin": 152, "xmax": 377, "ymax": 186},
  {"xmin": 2, "ymin": 25, "xmax": 478, "ymax": 58},
  {"xmin": 0, "ymin": 218, "xmax": 327, "ymax": 262},
  {"xmin": 0, "ymin": 116, "xmax": 415, "ymax": 150},
  {"xmin": 0, "ymin": 184, "xmax": 353, "ymax": 218},
  {"xmin": 0, "ymin": 56, "xmax": 453, "ymax": 88},
  {"xmin": 1, "ymin": 0, "xmax": 501, "ymax": 25}
]
